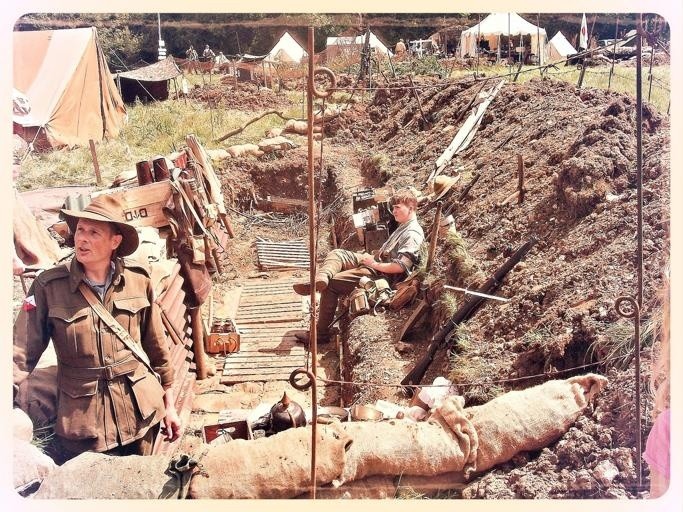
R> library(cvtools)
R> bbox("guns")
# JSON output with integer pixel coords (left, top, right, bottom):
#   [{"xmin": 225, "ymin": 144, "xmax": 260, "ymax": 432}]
[{"xmin": 400, "ymin": 241, "xmax": 535, "ymax": 400}]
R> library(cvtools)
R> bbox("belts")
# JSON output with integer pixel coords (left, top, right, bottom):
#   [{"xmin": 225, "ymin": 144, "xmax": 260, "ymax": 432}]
[{"xmin": 58, "ymin": 354, "xmax": 140, "ymax": 381}]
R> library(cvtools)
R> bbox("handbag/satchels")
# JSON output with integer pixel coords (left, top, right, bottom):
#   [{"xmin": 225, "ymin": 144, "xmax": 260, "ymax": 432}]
[
  {"xmin": 188, "ymin": 233, "xmax": 206, "ymax": 266},
  {"xmin": 388, "ymin": 271, "xmax": 421, "ymax": 313}
]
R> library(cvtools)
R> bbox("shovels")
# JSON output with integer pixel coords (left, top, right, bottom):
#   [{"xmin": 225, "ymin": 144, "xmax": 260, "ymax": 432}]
[
  {"xmin": 407, "ymin": 75, "xmax": 433, "ymax": 129},
  {"xmin": 400, "ymin": 201, "xmax": 443, "ymax": 339}
]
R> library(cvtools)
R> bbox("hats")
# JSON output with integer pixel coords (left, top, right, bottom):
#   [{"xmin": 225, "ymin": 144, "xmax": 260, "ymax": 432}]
[
  {"xmin": 60, "ymin": 193, "xmax": 140, "ymax": 258},
  {"xmin": 427, "ymin": 173, "xmax": 461, "ymax": 203}
]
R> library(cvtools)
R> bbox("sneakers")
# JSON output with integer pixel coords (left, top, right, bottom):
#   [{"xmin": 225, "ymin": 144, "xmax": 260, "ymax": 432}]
[
  {"xmin": 292, "ymin": 273, "xmax": 329, "ymax": 296},
  {"xmin": 294, "ymin": 329, "xmax": 331, "ymax": 344}
]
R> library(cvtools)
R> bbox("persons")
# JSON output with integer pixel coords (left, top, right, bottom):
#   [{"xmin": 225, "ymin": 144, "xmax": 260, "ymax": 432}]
[
  {"xmin": 202, "ymin": 44, "xmax": 215, "ymax": 75},
  {"xmin": 216, "ymin": 51, "xmax": 230, "ymax": 75},
  {"xmin": 184, "ymin": 45, "xmax": 199, "ymax": 75},
  {"xmin": 394, "ymin": 39, "xmax": 406, "ymax": 56},
  {"xmin": 12, "ymin": 188, "xmax": 181, "ymax": 460},
  {"xmin": 290, "ymin": 187, "xmax": 425, "ymax": 348}
]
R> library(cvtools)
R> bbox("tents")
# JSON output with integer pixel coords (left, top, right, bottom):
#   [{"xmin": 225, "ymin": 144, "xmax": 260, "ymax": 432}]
[
  {"xmin": 256, "ymin": 31, "xmax": 309, "ymax": 71},
  {"xmin": 543, "ymin": 31, "xmax": 578, "ymax": 64},
  {"xmin": 12, "ymin": 25, "xmax": 129, "ymax": 159},
  {"xmin": 460, "ymin": 14, "xmax": 546, "ymax": 67},
  {"xmin": 354, "ymin": 29, "xmax": 393, "ymax": 61}
]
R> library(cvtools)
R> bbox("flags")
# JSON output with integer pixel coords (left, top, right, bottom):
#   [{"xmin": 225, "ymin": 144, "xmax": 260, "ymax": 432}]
[{"xmin": 577, "ymin": 14, "xmax": 589, "ymax": 50}]
